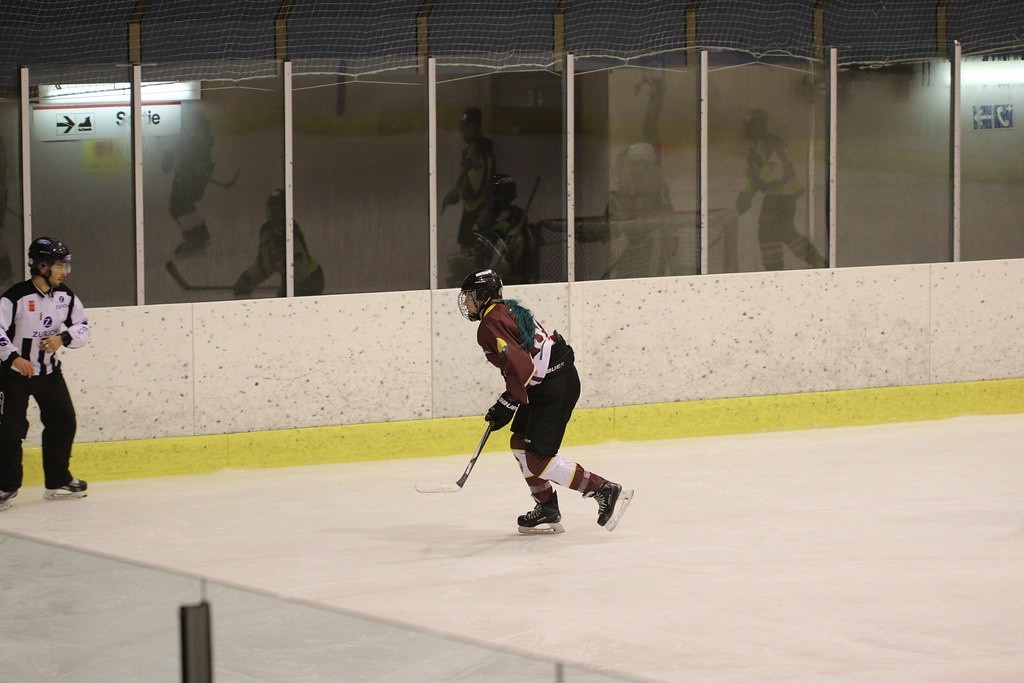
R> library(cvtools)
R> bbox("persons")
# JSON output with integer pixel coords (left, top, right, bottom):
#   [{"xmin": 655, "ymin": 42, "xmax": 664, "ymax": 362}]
[
  {"xmin": 163, "ymin": 111, "xmax": 211, "ymax": 257},
  {"xmin": 737, "ymin": 108, "xmax": 829, "ymax": 271},
  {"xmin": 457, "ymin": 271, "xmax": 633, "ymax": 536},
  {"xmin": 0, "ymin": 237, "xmax": 91, "ymax": 510},
  {"xmin": 441, "ymin": 109, "xmax": 497, "ymax": 251},
  {"xmin": 234, "ymin": 189, "xmax": 323, "ymax": 295},
  {"xmin": 578, "ymin": 144, "xmax": 679, "ymax": 281},
  {"xmin": 471, "ymin": 170, "xmax": 541, "ymax": 285}
]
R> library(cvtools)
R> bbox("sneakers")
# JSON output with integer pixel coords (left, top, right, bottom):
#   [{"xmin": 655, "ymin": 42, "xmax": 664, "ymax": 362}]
[
  {"xmin": 0, "ymin": 490, "xmax": 19, "ymax": 511},
  {"xmin": 171, "ymin": 234, "xmax": 212, "ymax": 258},
  {"xmin": 582, "ymin": 480, "xmax": 634, "ymax": 531},
  {"xmin": 41, "ymin": 479, "xmax": 88, "ymax": 499},
  {"xmin": 517, "ymin": 489, "xmax": 565, "ymax": 534}
]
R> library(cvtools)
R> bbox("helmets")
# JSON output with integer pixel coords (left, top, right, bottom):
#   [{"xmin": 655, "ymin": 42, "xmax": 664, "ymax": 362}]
[
  {"xmin": 27, "ymin": 237, "xmax": 73, "ymax": 276},
  {"xmin": 459, "ymin": 106, "xmax": 482, "ymax": 122},
  {"xmin": 742, "ymin": 109, "xmax": 768, "ymax": 124},
  {"xmin": 457, "ymin": 268, "xmax": 504, "ymax": 322},
  {"xmin": 625, "ymin": 142, "xmax": 656, "ymax": 162}
]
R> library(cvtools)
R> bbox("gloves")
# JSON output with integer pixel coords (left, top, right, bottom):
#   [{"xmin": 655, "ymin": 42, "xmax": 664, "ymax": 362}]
[{"xmin": 485, "ymin": 391, "xmax": 521, "ymax": 431}]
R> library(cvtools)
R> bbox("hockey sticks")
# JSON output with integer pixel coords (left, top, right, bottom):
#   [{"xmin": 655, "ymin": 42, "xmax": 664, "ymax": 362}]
[
  {"xmin": 169, "ymin": 166, "xmax": 241, "ymax": 187},
  {"xmin": 417, "ymin": 423, "xmax": 492, "ymax": 493},
  {"xmin": 524, "ymin": 176, "xmax": 541, "ymax": 216},
  {"xmin": 167, "ymin": 262, "xmax": 281, "ymax": 295},
  {"xmin": 441, "ymin": 200, "xmax": 448, "ymax": 213}
]
